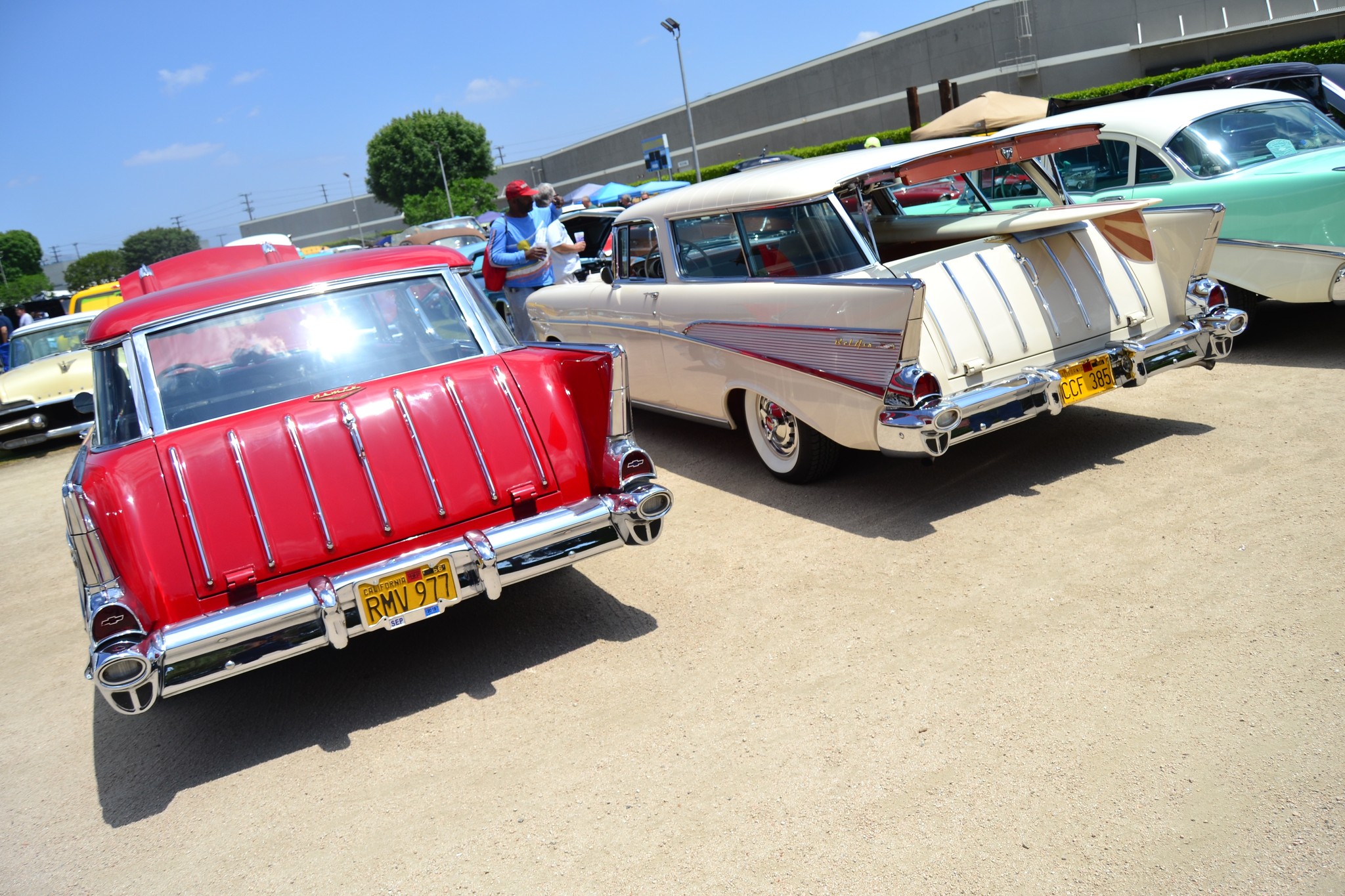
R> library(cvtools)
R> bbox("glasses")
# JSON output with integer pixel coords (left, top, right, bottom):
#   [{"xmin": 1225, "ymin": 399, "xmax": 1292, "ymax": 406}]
[{"xmin": 642, "ymin": 198, "xmax": 647, "ymax": 201}]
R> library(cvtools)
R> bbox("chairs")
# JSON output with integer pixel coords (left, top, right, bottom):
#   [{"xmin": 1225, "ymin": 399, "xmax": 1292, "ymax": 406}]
[{"xmin": 775, "ymin": 223, "xmax": 836, "ymax": 263}]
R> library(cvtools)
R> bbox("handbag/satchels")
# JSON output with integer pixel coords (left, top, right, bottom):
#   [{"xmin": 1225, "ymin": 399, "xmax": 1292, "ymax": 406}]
[{"xmin": 481, "ymin": 216, "xmax": 507, "ymax": 292}]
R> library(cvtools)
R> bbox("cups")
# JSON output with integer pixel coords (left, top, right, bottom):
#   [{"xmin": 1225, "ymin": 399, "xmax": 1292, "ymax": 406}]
[
  {"xmin": 535, "ymin": 242, "xmax": 548, "ymax": 259},
  {"xmin": 574, "ymin": 232, "xmax": 584, "ymax": 242}
]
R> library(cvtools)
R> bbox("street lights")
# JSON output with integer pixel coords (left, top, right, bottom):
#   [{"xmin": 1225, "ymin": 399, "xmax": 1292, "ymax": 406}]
[
  {"xmin": 342, "ymin": 171, "xmax": 365, "ymax": 247},
  {"xmin": 530, "ymin": 166, "xmax": 536, "ymax": 188},
  {"xmin": 430, "ymin": 140, "xmax": 455, "ymax": 218},
  {"xmin": 537, "ymin": 168, "xmax": 542, "ymax": 184},
  {"xmin": 661, "ymin": 17, "xmax": 702, "ymax": 183}
]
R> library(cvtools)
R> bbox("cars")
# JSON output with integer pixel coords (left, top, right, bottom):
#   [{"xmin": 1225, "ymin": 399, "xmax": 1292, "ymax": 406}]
[
  {"xmin": 522, "ymin": 120, "xmax": 1248, "ymax": 487},
  {"xmin": 60, "ymin": 244, "xmax": 674, "ymax": 716},
  {"xmin": 838, "ymin": 61, "xmax": 1345, "ymax": 353},
  {"xmin": 1, "ymin": 153, "xmax": 807, "ymax": 464}
]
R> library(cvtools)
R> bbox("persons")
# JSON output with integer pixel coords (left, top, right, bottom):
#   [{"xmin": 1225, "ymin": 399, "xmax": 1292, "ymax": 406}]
[
  {"xmin": 582, "ymin": 196, "xmax": 598, "ymax": 209},
  {"xmin": 0, "ymin": 304, "xmax": 34, "ymax": 372},
  {"xmin": 488, "ymin": 180, "xmax": 555, "ymax": 342},
  {"xmin": 623, "ymin": 195, "xmax": 634, "ymax": 208},
  {"xmin": 858, "ymin": 137, "xmax": 894, "ymax": 212},
  {"xmin": 641, "ymin": 192, "xmax": 649, "ymax": 201},
  {"xmin": 535, "ymin": 182, "xmax": 586, "ymax": 285}
]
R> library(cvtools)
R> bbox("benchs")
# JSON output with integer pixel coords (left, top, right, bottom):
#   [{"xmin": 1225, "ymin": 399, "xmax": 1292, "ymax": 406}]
[{"xmin": 119, "ymin": 338, "xmax": 464, "ymax": 442}]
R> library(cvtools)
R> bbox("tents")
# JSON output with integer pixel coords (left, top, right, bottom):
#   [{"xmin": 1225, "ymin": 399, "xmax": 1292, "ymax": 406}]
[
  {"xmin": 562, "ymin": 181, "xmax": 691, "ymax": 207},
  {"xmin": 476, "ymin": 211, "xmax": 504, "ymax": 224},
  {"xmin": 910, "ymin": 91, "xmax": 1049, "ymax": 142}
]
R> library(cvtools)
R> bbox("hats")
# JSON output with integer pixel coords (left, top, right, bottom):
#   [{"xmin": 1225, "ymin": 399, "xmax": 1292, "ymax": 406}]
[
  {"xmin": 505, "ymin": 180, "xmax": 539, "ymax": 198},
  {"xmin": 865, "ymin": 137, "xmax": 881, "ymax": 148}
]
